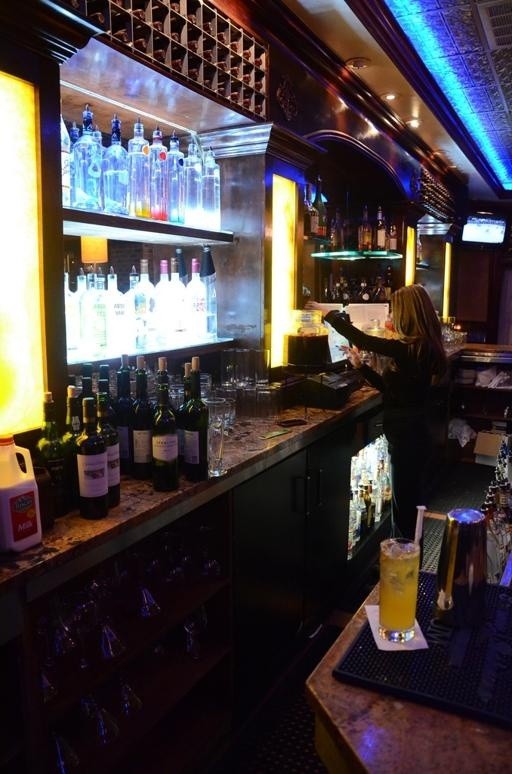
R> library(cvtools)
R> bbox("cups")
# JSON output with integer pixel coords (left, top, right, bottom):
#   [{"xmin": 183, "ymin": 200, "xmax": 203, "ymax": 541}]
[
  {"xmin": 379, "ymin": 533, "xmax": 418, "ymax": 644},
  {"xmin": 436, "ymin": 507, "xmax": 492, "ymax": 629},
  {"xmin": 441, "ymin": 316, "xmax": 467, "ymax": 353},
  {"xmin": 214, "ymin": 348, "xmax": 286, "ymax": 420}
]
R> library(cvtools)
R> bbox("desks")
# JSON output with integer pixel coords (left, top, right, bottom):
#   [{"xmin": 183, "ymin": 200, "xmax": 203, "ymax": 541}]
[{"xmin": 304, "ymin": 563, "xmax": 510, "ymax": 771}]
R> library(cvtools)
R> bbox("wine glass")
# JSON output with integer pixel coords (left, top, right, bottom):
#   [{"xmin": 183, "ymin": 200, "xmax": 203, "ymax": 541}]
[{"xmin": 208, "ymin": 395, "xmax": 236, "ymax": 479}]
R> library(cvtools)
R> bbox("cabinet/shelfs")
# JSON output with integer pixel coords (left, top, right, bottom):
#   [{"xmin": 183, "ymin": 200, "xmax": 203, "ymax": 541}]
[
  {"xmin": 448, "ymin": 351, "xmax": 511, "ymax": 468},
  {"xmin": 305, "ymin": 173, "xmax": 400, "ymax": 342},
  {"xmin": 12, "ymin": 501, "xmax": 228, "ymax": 771},
  {"xmin": 233, "ymin": 422, "xmax": 360, "ymax": 656},
  {"xmin": 38, "ymin": 84, "xmax": 270, "ymax": 380}
]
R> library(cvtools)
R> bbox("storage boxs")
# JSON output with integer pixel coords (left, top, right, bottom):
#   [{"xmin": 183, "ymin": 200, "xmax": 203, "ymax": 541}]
[{"xmin": 473, "ymin": 430, "xmax": 507, "ymax": 457}]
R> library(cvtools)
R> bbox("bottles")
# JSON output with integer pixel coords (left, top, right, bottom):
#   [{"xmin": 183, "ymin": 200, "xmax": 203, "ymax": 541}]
[
  {"xmin": 32, "ymin": 352, "xmax": 209, "ymax": 524},
  {"xmin": 348, "ymin": 435, "xmax": 393, "ymax": 558},
  {"xmin": 60, "ymin": 101, "xmax": 222, "ymax": 232},
  {"xmin": 49, "ymin": 0, "xmax": 264, "ymax": 117},
  {"xmin": 300, "ymin": 177, "xmax": 397, "ymax": 253},
  {"xmin": 64, "ymin": 242, "xmax": 217, "ymax": 357},
  {"xmin": 331, "ymin": 262, "xmax": 393, "ymax": 303}
]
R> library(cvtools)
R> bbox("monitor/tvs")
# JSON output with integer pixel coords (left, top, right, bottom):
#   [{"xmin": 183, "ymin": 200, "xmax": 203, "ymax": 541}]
[
  {"xmin": 322, "ymin": 313, "xmax": 353, "ymax": 369},
  {"xmin": 458, "ymin": 213, "xmax": 510, "ymax": 248}
]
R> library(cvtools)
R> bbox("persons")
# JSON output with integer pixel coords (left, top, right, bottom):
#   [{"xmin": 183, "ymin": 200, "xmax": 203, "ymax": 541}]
[{"xmin": 305, "ymin": 286, "xmax": 448, "ymax": 569}]
